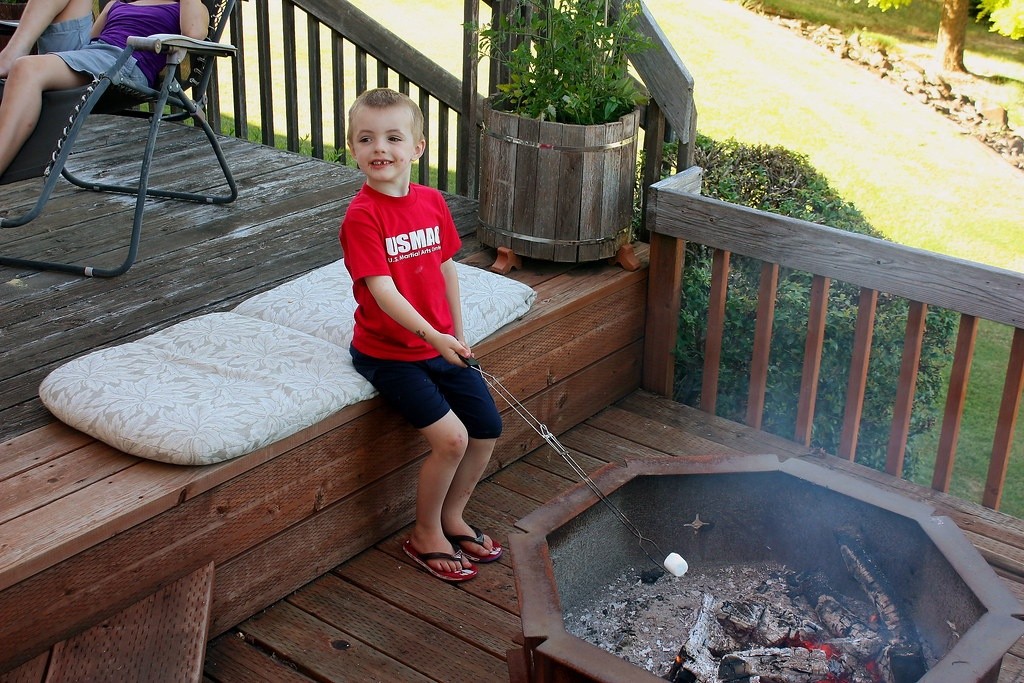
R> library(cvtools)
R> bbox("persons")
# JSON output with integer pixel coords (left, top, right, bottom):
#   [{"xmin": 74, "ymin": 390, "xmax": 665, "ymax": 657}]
[
  {"xmin": 338, "ymin": 88, "xmax": 503, "ymax": 582},
  {"xmin": 0, "ymin": 0, "xmax": 211, "ymax": 174}
]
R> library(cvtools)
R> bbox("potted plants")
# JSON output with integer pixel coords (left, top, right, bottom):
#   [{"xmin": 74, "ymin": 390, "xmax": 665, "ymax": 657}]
[{"xmin": 459, "ymin": 0, "xmax": 664, "ymax": 262}]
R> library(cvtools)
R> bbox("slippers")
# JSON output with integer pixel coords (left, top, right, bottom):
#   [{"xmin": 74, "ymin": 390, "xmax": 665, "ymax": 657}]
[
  {"xmin": 403, "ymin": 538, "xmax": 478, "ymax": 581},
  {"xmin": 443, "ymin": 525, "xmax": 502, "ymax": 561}
]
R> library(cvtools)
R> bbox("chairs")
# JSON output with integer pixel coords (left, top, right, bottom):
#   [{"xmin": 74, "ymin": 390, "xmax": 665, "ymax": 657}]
[{"xmin": 0, "ymin": 0, "xmax": 238, "ymax": 280}]
